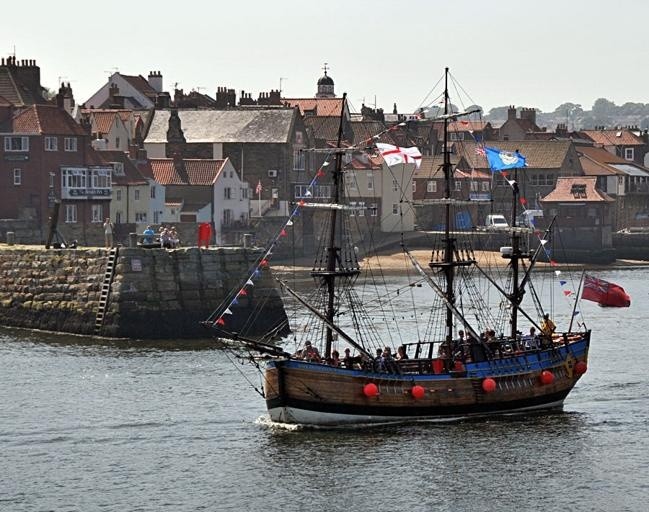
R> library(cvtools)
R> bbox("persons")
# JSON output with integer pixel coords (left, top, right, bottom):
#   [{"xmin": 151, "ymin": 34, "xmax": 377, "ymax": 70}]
[
  {"xmin": 103, "ymin": 217, "xmax": 115, "ymax": 249},
  {"xmin": 299, "ymin": 340, "xmax": 410, "ymax": 374},
  {"xmin": 524, "ymin": 326, "xmax": 540, "ymax": 347},
  {"xmin": 142, "ymin": 226, "xmax": 180, "ymax": 249},
  {"xmin": 440, "ymin": 329, "xmax": 500, "ymax": 363},
  {"xmin": 540, "ymin": 314, "xmax": 557, "ymax": 343}
]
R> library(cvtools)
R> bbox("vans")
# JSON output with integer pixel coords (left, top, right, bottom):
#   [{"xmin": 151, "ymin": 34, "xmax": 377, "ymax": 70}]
[{"xmin": 485, "ymin": 215, "xmax": 509, "ymax": 231}]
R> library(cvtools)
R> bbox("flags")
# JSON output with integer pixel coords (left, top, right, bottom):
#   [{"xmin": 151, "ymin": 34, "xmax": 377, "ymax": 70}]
[
  {"xmin": 375, "ymin": 141, "xmax": 423, "ymax": 169},
  {"xmin": 483, "ymin": 146, "xmax": 529, "ymax": 173},
  {"xmin": 255, "ymin": 182, "xmax": 265, "ymax": 194},
  {"xmin": 579, "ymin": 274, "xmax": 630, "ymax": 308}
]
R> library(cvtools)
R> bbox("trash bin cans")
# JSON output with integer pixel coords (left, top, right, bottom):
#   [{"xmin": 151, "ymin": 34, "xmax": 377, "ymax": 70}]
[
  {"xmin": 244, "ymin": 234, "xmax": 253, "ymax": 248},
  {"xmin": 7, "ymin": 231, "xmax": 14, "ymax": 246}
]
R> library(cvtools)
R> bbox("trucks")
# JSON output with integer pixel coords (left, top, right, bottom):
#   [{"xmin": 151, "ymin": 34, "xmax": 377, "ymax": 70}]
[{"xmin": 515, "ymin": 210, "xmax": 543, "ymax": 227}]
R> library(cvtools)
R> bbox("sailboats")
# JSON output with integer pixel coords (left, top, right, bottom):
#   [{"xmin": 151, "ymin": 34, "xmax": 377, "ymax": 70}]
[{"xmin": 198, "ymin": 66, "xmax": 592, "ymax": 424}]
